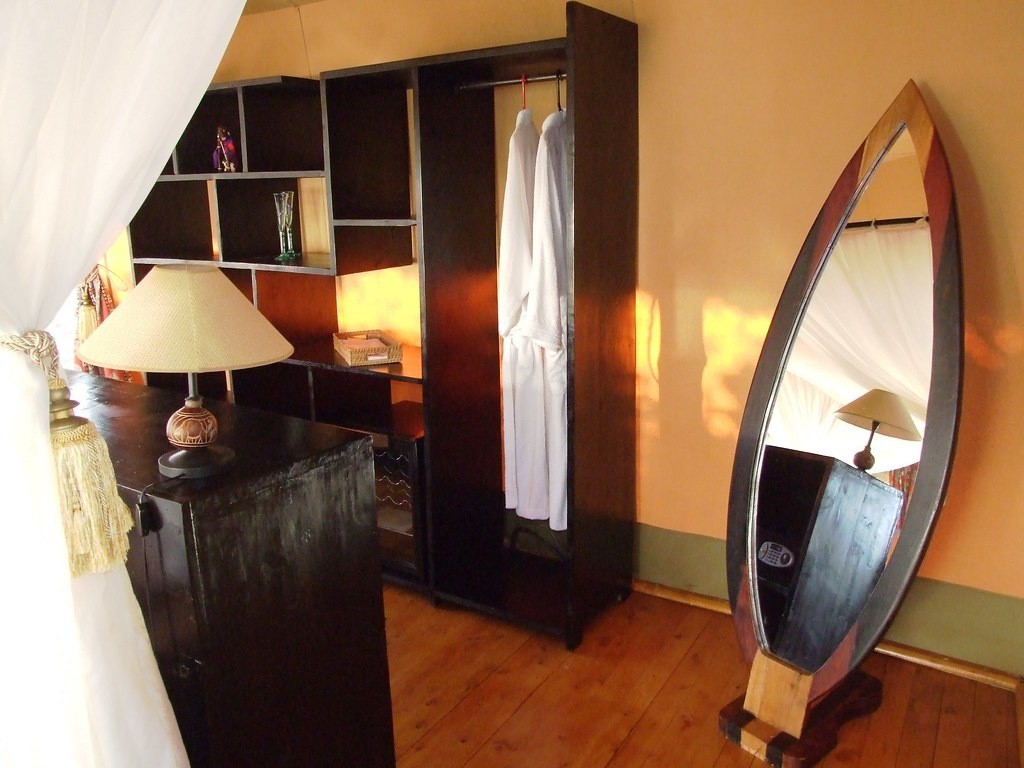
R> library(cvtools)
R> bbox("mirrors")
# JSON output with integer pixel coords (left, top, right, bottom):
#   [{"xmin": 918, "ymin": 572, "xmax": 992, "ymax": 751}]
[{"xmin": 717, "ymin": 78, "xmax": 965, "ymax": 768}]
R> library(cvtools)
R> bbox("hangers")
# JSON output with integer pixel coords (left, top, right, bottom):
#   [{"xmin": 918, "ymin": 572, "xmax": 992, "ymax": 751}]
[
  {"xmin": 555, "ymin": 68, "xmax": 564, "ymax": 112},
  {"xmin": 521, "ymin": 72, "xmax": 528, "ymax": 110}
]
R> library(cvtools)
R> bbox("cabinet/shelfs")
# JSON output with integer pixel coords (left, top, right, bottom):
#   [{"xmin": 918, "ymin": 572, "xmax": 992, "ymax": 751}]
[
  {"xmin": 757, "ymin": 445, "xmax": 904, "ymax": 672},
  {"xmin": 63, "ymin": 368, "xmax": 396, "ymax": 768},
  {"xmin": 126, "ymin": 0, "xmax": 637, "ymax": 652}
]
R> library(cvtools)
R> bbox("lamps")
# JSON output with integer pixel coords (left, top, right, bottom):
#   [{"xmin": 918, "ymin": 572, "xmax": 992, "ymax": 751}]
[
  {"xmin": 75, "ymin": 262, "xmax": 294, "ymax": 480},
  {"xmin": 831, "ymin": 388, "xmax": 922, "ymax": 471}
]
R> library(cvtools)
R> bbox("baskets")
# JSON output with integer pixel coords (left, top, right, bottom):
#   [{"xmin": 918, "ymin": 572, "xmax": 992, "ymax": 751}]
[{"xmin": 332, "ymin": 329, "xmax": 403, "ymax": 367}]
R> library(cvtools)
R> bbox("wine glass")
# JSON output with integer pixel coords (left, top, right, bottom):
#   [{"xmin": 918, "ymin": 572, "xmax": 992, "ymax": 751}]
[
  {"xmin": 283, "ymin": 191, "xmax": 301, "ymax": 257},
  {"xmin": 272, "ymin": 193, "xmax": 294, "ymax": 261}
]
